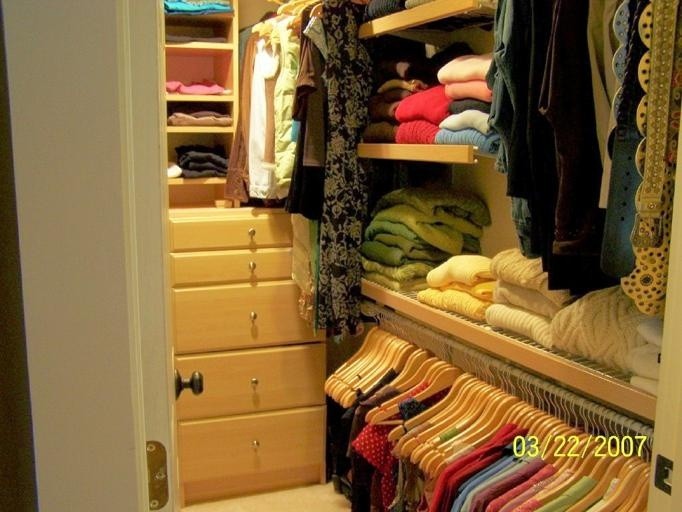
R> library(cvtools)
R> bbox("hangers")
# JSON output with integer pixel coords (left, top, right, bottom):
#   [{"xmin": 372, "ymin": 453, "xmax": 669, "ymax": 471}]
[{"xmin": 277, "ymin": 0, "xmax": 331, "ymax": 18}]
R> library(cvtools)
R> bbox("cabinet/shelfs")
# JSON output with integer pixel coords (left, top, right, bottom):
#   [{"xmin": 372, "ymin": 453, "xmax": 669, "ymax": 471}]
[
  {"xmin": 163, "ymin": 0, "xmax": 328, "ymax": 506},
  {"xmin": 356, "ymin": 0, "xmax": 657, "ymax": 419}
]
